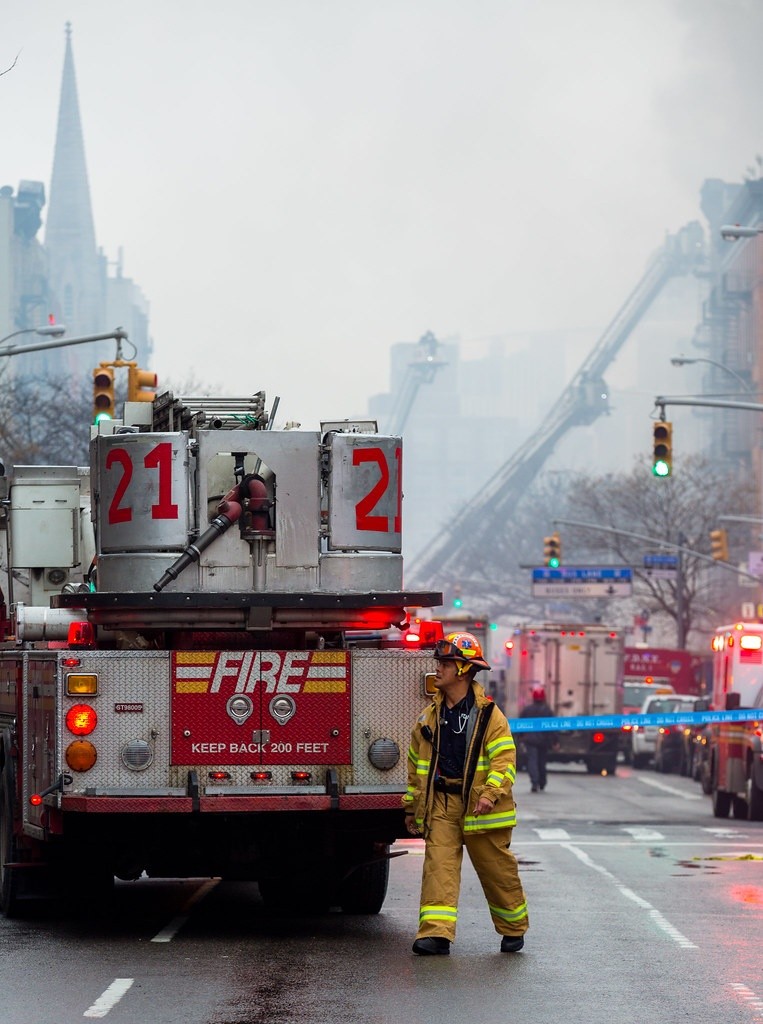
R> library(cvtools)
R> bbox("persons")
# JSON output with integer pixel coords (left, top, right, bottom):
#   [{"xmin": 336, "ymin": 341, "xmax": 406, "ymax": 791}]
[
  {"xmin": 517, "ymin": 688, "xmax": 561, "ymax": 791},
  {"xmin": 402, "ymin": 632, "xmax": 530, "ymax": 953}
]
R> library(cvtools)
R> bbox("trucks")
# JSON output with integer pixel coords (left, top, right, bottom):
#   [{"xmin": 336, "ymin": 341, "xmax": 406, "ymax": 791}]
[{"xmin": 506, "ymin": 620, "xmax": 624, "ymax": 775}]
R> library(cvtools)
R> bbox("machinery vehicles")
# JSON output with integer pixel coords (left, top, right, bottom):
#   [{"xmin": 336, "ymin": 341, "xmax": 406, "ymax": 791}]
[
  {"xmin": 388, "ymin": 332, "xmax": 467, "ymax": 436},
  {"xmin": 403, "ymin": 218, "xmax": 708, "ymax": 607}
]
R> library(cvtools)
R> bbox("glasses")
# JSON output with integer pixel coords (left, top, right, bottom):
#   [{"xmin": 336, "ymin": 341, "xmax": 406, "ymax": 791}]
[{"xmin": 434, "ymin": 640, "xmax": 463, "ymax": 658}]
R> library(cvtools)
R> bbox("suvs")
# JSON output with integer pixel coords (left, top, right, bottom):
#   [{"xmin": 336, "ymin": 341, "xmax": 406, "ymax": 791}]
[{"xmin": 632, "ymin": 694, "xmax": 703, "ymax": 769}]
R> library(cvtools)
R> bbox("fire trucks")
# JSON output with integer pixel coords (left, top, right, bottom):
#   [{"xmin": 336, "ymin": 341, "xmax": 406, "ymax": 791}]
[
  {"xmin": 621, "ymin": 647, "xmax": 708, "ymax": 766},
  {"xmin": 0, "ymin": 386, "xmax": 445, "ymax": 916},
  {"xmin": 709, "ymin": 602, "xmax": 763, "ymax": 821}
]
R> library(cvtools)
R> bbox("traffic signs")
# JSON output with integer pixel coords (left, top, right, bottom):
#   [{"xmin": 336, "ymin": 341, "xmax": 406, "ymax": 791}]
[{"xmin": 531, "ymin": 570, "xmax": 632, "ymax": 600}]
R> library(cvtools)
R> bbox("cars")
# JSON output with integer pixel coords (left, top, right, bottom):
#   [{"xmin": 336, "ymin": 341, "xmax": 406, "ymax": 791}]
[
  {"xmin": 743, "ymin": 684, "xmax": 763, "ymax": 819},
  {"xmin": 657, "ymin": 698, "xmax": 714, "ymax": 780}
]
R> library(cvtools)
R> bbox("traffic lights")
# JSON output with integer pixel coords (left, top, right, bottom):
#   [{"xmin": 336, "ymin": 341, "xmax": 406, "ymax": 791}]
[
  {"xmin": 545, "ymin": 538, "xmax": 560, "ymax": 568},
  {"xmin": 129, "ymin": 363, "xmax": 158, "ymax": 402},
  {"xmin": 710, "ymin": 532, "xmax": 728, "ymax": 561},
  {"xmin": 653, "ymin": 425, "xmax": 672, "ymax": 477},
  {"xmin": 94, "ymin": 368, "xmax": 114, "ymax": 426}
]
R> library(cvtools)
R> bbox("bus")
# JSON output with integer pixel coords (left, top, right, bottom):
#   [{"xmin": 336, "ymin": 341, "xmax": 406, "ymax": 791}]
[{"xmin": 436, "ymin": 613, "xmax": 490, "ymax": 698}]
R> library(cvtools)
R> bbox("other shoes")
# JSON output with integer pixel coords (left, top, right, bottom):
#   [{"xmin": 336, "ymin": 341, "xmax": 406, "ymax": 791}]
[
  {"xmin": 412, "ymin": 935, "xmax": 451, "ymax": 956},
  {"xmin": 501, "ymin": 931, "xmax": 523, "ymax": 952}
]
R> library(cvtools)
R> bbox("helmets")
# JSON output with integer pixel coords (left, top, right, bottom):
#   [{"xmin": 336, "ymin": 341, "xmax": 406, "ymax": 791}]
[
  {"xmin": 432, "ymin": 632, "xmax": 491, "ymax": 669},
  {"xmin": 532, "ymin": 689, "xmax": 546, "ymax": 702}
]
